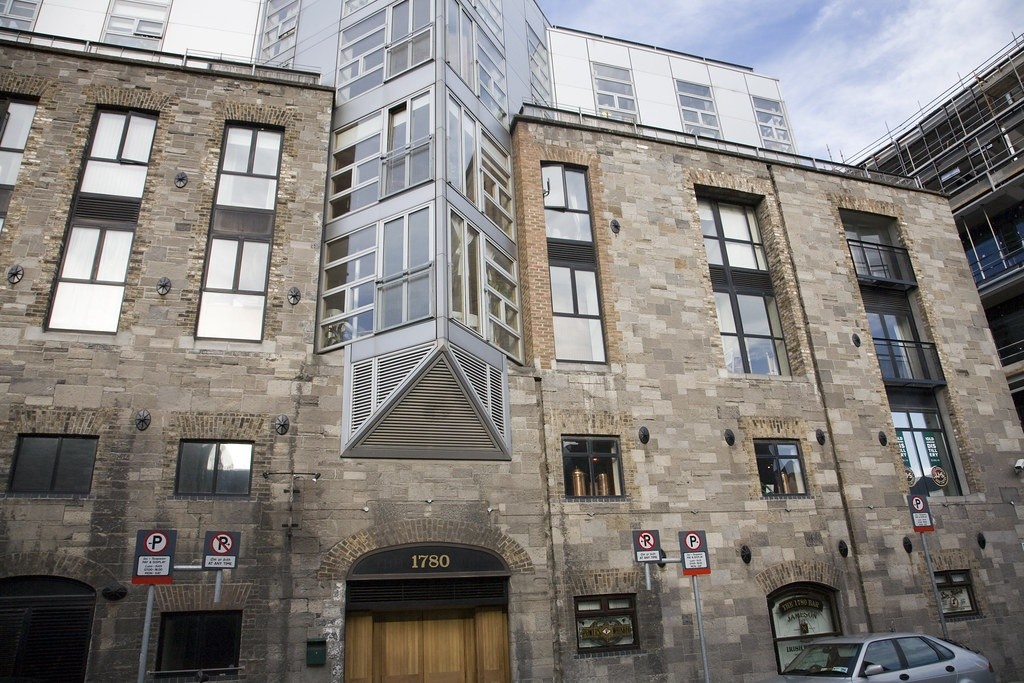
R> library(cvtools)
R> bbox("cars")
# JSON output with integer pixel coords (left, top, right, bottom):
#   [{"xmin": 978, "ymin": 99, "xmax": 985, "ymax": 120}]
[{"xmin": 755, "ymin": 633, "xmax": 1001, "ymax": 683}]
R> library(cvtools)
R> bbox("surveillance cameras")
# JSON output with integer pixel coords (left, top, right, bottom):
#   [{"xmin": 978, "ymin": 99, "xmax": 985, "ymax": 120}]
[{"xmin": 1014, "ymin": 459, "xmax": 1024, "ymax": 469}]
